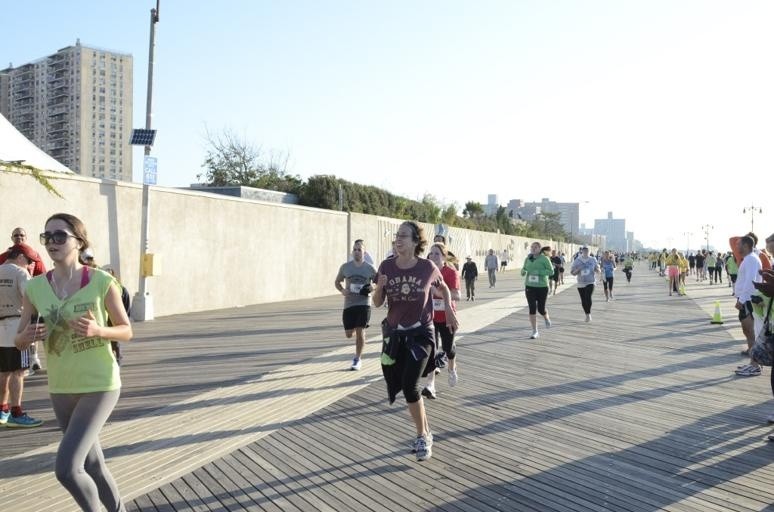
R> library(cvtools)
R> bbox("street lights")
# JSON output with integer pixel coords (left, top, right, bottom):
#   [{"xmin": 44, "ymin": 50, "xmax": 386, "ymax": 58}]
[
  {"xmin": 702, "ymin": 223, "xmax": 714, "ymax": 251},
  {"xmin": 570, "ymin": 201, "xmax": 589, "ymax": 261},
  {"xmin": 682, "ymin": 231, "xmax": 694, "ymax": 249},
  {"xmin": 742, "ymin": 201, "xmax": 763, "ymax": 233}
]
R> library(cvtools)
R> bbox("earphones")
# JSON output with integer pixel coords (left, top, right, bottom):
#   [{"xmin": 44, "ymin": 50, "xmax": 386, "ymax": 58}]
[{"xmin": 76, "ymin": 244, "xmax": 80, "ymax": 248}]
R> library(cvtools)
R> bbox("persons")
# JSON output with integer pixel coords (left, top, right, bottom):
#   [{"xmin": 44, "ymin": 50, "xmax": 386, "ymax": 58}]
[
  {"xmin": 734, "ymin": 235, "xmax": 763, "ymax": 376},
  {"xmin": 462, "ymin": 248, "xmax": 509, "ymax": 302},
  {"xmin": 349, "ymin": 235, "xmax": 459, "ymax": 277},
  {"xmin": 0, "ymin": 227, "xmax": 47, "ymax": 378},
  {"xmin": 371, "ymin": 220, "xmax": 455, "ymax": 461},
  {"xmin": 420, "ymin": 242, "xmax": 460, "ymax": 400},
  {"xmin": 0, "ymin": 242, "xmax": 44, "ymax": 428},
  {"xmin": 79, "ymin": 247, "xmax": 130, "ymax": 363},
  {"xmin": 758, "ymin": 234, "xmax": 774, "ymax": 442},
  {"xmin": 333, "ymin": 243, "xmax": 377, "ymax": 372},
  {"xmin": 520, "ymin": 241, "xmax": 641, "ymax": 338},
  {"xmin": 13, "ymin": 214, "xmax": 134, "ymax": 512},
  {"xmin": 642, "ymin": 248, "xmax": 739, "ymax": 296}
]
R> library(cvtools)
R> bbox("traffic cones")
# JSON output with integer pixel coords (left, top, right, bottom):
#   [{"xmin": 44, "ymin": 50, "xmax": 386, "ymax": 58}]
[
  {"xmin": 680, "ymin": 283, "xmax": 687, "ymax": 296},
  {"xmin": 708, "ymin": 300, "xmax": 724, "ymax": 324}
]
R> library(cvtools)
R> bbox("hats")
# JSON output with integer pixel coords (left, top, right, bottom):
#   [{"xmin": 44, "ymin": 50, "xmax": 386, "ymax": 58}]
[{"xmin": 8, "ymin": 243, "xmax": 41, "ymax": 263}]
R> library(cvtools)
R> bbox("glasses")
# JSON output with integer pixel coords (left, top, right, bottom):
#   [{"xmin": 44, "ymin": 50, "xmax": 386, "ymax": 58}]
[{"xmin": 39, "ymin": 230, "xmax": 82, "ymax": 247}]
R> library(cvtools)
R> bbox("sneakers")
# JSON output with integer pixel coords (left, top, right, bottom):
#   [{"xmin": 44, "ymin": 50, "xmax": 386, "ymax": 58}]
[
  {"xmin": 544, "ymin": 319, "xmax": 552, "ymax": 330},
  {"xmin": 7, "ymin": 414, "xmax": 45, "ymax": 429},
  {"xmin": 447, "ymin": 365, "xmax": 458, "ymax": 388},
  {"xmin": 767, "ymin": 413, "xmax": 774, "ymax": 422},
  {"xmin": 0, "ymin": 409, "xmax": 11, "ymax": 424},
  {"xmin": 734, "ymin": 348, "xmax": 764, "ymax": 377},
  {"xmin": 768, "ymin": 435, "xmax": 774, "ymax": 441},
  {"xmin": 585, "ymin": 313, "xmax": 591, "ymax": 322},
  {"xmin": 530, "ymin": 329, "xmax": 539, "ymax": 338},
  {"xmin": 411, "ymin": 430, "xmax": 434, "ymax": 462},
  {"xmin": 350, "ymin": 357, "xmax": 362, "ymax": 371},
  {"xmin": 421, "ymin": 383, "xmax": 438, "ymax": 400}
]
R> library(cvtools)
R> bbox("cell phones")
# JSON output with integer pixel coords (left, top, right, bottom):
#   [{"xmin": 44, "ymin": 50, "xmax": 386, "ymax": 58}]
[
  {"xmin": 752, "ymin": 280, "xmax": 756, "ymax": 286},
  {"xmin": 31, "ymin": 314, "xmax": 45, "ymax": 342}
]
